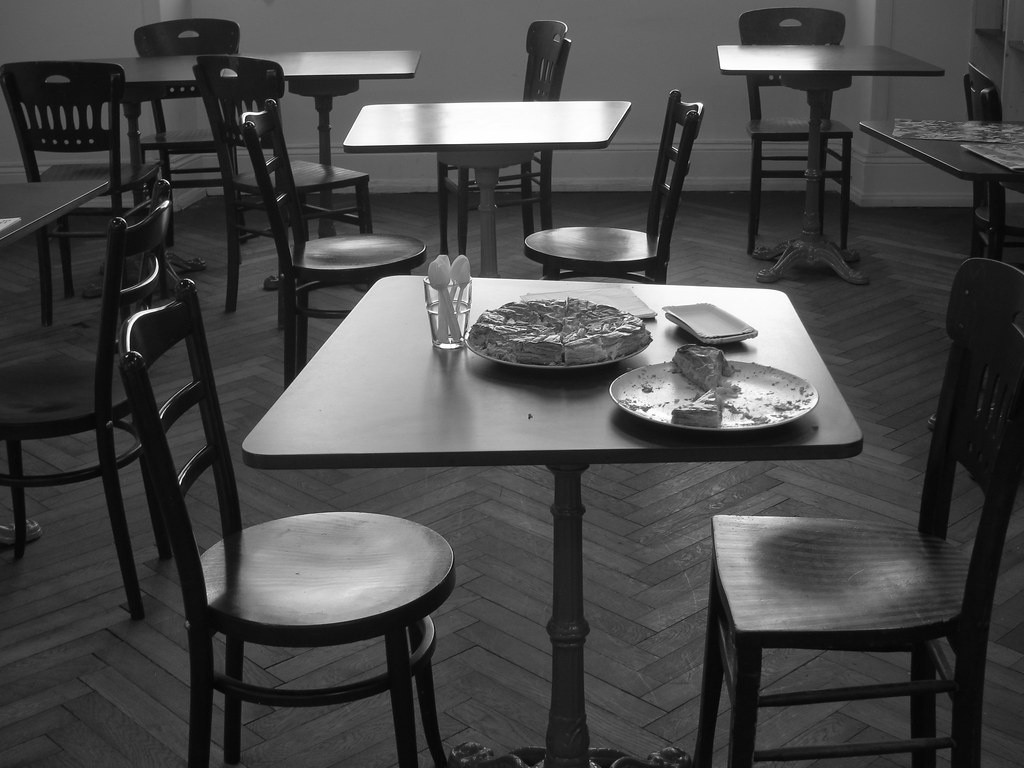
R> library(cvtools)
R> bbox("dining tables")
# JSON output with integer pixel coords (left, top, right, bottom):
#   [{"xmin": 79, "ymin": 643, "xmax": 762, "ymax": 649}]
[
  {"xmin": 0, "ymin": 172, "xmax": 114, "ymax": 247},
  {"xmin": 237, "ymin": 257, "xmax": 864, "ymax": 768},
  {"xmin": 716, "ymin": 39, "xmax": 949, "ymax": 287},
  {"xmin": 77, "ymin": 47, "xmax": 420, "ymax": 248},
  {"xmin": 342, "ymin": 100, "xmax": 633, "ymax": 279},
  {"xmin": 859, "ymin": 112, "xmax": 1023, "ymax": 266}
]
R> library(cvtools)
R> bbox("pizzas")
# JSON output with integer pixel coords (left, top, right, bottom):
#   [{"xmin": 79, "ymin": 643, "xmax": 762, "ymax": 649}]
[
  {"xmin": 671, "ymin": 345, "xmax": 743, "ymax": 426},
  {"xmin": 472, "ymin": 295, "xmax": 651, "ymax": 364}
]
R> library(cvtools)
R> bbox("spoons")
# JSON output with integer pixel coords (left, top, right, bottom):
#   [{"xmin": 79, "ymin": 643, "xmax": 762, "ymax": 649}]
[{"xmin": 428, "ymin": 253, "xmax": 471, "ymax": 345}]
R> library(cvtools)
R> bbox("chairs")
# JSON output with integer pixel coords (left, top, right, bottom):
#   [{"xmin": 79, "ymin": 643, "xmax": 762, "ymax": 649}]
[
  {"xmin": 0, "ymin": 57, "xmax": 169, "ymax": 322},
  {"xmin": 1, "ymin": 170, "xmax": 179, "ymax": 566},
  {"xmin": 128, "ymin": 17, "xmax": 246, "ymax": 227},
  {"xmin": 521, "ymin": 87, "xmax": 704, "ymax": 282},
  {"xmin": 238, "ymin": 102, "xmax": 428, "ymax": 394},
  {"xmin": 960, "ymin": 65, "xmax": 1024, "ymax": 272},
  {"xmin": 438, "ymin": 17, "xmax": 576, "ymax": 254},
  {"xmin": 116, "ymin": 282, "xmax": 458, "ymax": 768},
  {"xmin": 735, "ymin": 7, "xmax": 862, "ymax": 268},
  {"xmin": 690, "ymin": 256, "xmax": 1024, "ymax": 768},
  {"xmin": 194, "ymin": 54, "xmax": 378, "ymax": 315}
]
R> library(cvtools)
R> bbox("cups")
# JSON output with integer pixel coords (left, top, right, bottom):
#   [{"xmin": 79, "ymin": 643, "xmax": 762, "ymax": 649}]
[{"xmin": 422, "ymin": 274, "xmax": 474, "ymax": 351}]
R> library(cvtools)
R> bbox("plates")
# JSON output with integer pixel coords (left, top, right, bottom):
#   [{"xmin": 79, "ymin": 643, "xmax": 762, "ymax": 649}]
[
  {"xmin": 460, "ymin": 324, "xmax": 652, "ymax": 370},
  {"xmin": 608, "ymin": 360, "xmax": 820, "ymax": 431},
  {"xmin": 664, "ymin": 312, "xmax": 758, "ymax": 346},
  {"xmin": 660, "ymin": 303, "xmax": 755, "ymax": 339}
]
